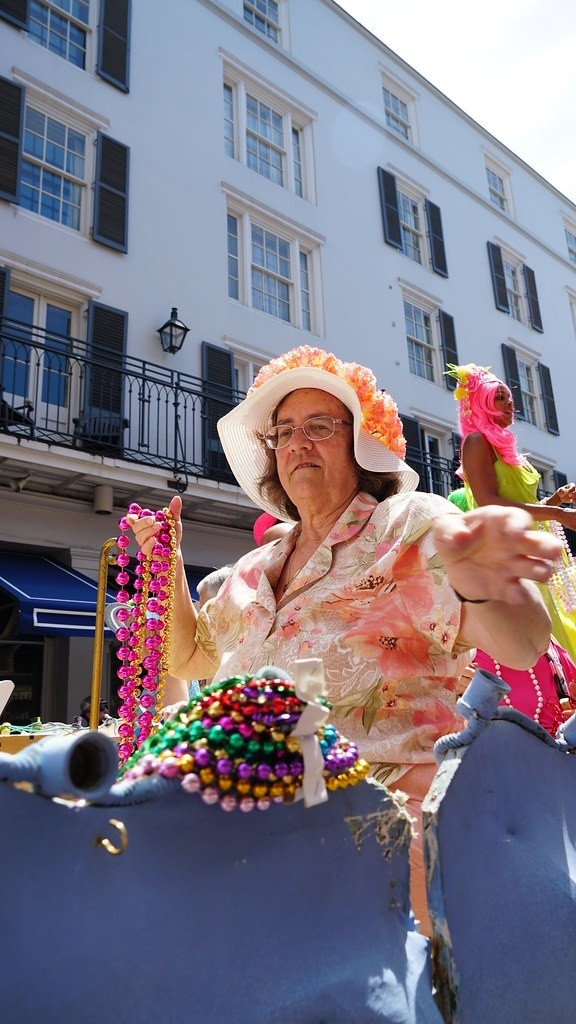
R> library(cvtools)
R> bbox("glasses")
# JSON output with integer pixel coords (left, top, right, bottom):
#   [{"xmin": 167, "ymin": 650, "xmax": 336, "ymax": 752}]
[
  {"xmin": 100, "ymin": 705, "xmax": 109, "ymax": 711},
  {"xmin": 259, "ymin": 415, "xmax": 352, "ymax": 449}
]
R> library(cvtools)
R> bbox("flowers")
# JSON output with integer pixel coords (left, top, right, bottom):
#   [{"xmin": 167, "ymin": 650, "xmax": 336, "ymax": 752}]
[
  {"xmin": 441, "ymin": 362, "xmax": 491, "ymax": 416},
  {"xmin": 247, "ymin": 345, "xmax": 407, "ymax": 461}
]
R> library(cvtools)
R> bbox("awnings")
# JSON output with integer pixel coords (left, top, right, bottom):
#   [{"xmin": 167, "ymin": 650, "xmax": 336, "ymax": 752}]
[
  {"xmin": 0, "ymin": 553, "xmax": 121, "ymax": 638},
  {"xmin": 123, "ymin": 563, "xmax": 218, "ymax": 602}
]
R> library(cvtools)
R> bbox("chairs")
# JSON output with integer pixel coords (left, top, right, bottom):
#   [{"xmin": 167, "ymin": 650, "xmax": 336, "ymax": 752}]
[
  {"xmin": 72, "ymin": 404, "xmax": 129, "ymax": 456},
  {"xmin": 0, "ymin": 383, "xmax": 36, "ymax": 437}
]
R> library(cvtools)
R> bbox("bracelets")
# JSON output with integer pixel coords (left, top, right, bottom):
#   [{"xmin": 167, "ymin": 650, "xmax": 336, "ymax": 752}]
[
  {"xmin": 547, "ymin": 493, "xmax": 560, "ymax": 507},
  {"xmin": 454, "ymin": 590, "xmax": 489, "ymax": 603}
]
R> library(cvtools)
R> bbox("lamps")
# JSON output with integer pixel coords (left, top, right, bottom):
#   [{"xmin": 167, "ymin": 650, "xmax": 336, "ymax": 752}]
[
  {"xmin": 93, "ymin": 485, "xmax": 114, "ymax": 516},
  {"xmin": 9, "ymin": 473, "xmax": 33, "ymax": 492},
  {"xmin": 157, "ymin": 307, "xmax": 190, "ymax": 353}
]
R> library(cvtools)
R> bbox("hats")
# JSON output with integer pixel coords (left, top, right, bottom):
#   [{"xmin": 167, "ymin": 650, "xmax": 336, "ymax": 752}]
[
  {"xmin": 217, "ymin": 345, "xmax": 420, "ymax": 525},
  {"xmin": 253, "ymin": 512, "xmax": 278, "ymax": 547},
  {"xmin": 81, "ymin": 695, "xmax": 107, "ymax": 714}
]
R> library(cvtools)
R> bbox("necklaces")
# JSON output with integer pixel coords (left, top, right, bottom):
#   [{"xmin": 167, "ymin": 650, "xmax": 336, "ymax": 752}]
[
  {"xmin": 538, "ymin": 497, "xmax": 576, "ymax": 614},
  {"xmin": 282, "ymin": 549, "xmax": 308, "ymax": 592},
  {"xmin": 492, "ymin": 657, "xmax": 543, "ymax": 724},
  {"xmin": 116, "ymin": 503, "xmax": 369, "ymax": 812}
]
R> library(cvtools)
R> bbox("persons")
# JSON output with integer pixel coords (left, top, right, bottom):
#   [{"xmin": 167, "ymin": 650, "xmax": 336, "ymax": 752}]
[
  {"xmin": 261, "ymin": 522, "xmax": 294, "ymax": 545},
  {"xmin": 473, "ymin": 636, "xmax": 576, "ymax": 739},
  {"xmin": 457, "ymin": 364, "xmax": 576, "ymax": 662},
  {"xmin": 132, "ymin": 347, "xmax": 564, "ymax": 943},
  {"xmin": 103, "ymin": 598, "xmax": 199, "ymax": 750},
  {"xmin": 80, "ymin": 695, "xmax": 110, "ymax": 725},
  {"xmin": 197, "ymin": 566, "xmax": 233, "ymax": 608}
]
